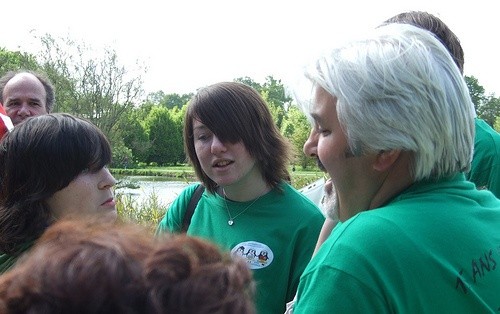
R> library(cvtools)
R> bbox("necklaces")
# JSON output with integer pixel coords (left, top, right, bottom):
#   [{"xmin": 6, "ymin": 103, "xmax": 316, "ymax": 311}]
[{"xmin": 222, "ymin": 186, "xmax": 270, "ymax": 226}]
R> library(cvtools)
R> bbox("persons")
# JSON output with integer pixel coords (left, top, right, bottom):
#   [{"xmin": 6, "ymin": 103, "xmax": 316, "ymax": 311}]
[
  {"xmin": 376, "ymin": 13, "xmax": 500, "ymax": 200},
  {"xmin": 0, "ymin": 218, "xmax": 256, "ymax": 314},
  {"xmin": 0, "ymin": 113, "xmax": 118, "ymax": 277},
  {"xmin": 154, "ymin": 81, "xmax": 328, "ymax": 314},
  {"xmin": 288, "ymin": 22, "xmax": 500, "ymax": 314},
  {"xmin": 0, "ymin": 70, "xmax": 55, "ymax": 127}
]
